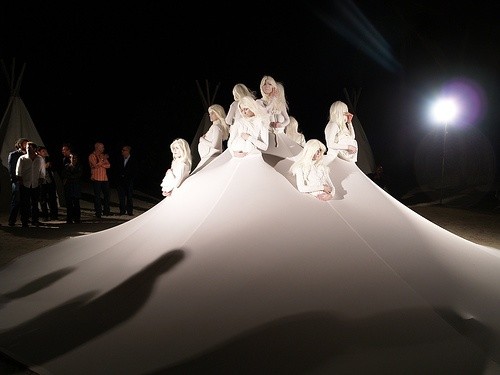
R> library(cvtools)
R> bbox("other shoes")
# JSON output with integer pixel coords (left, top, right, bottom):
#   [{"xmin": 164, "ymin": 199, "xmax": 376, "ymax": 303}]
[
  {"xmin": 120, "ymin": 210, "xmax": 125, "ymax": 214},
  {"xmin": 128, "ymin": 211, "xmax": 133, "ymax": 215}
]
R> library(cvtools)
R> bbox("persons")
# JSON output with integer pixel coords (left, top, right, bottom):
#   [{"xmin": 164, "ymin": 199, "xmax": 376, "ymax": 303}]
[
  {"xmin": 199, "ymin": 104, "xmax": 229, "ymax": 160},
  {"xmin": 160, "ymin": 138, "xmax": 192, "ymax": 196},
  {"xmin": 116, "ymin": 145, "xmax": 137, "ymax": 216},
  {"xmin": 9, "ymin": 137, "xmax": 60, "ymax": 226},
  {"xmin": 290, "ymin": 139, "xmax": 335, "ymax": 201},
  {"xmin": 61, "ymin": 144, "xmax": 83, "ymax": 225},
  {"xmin": 225, "ymin": 75, "xmax": 305, "ymax": 152},
  {"xmin": 89, "ymin": 143, "xmax": 113, "ymax": 219},
  {"xmin": 324, "ymin": 101, "xmax": 358, "ymax": 162}
]
